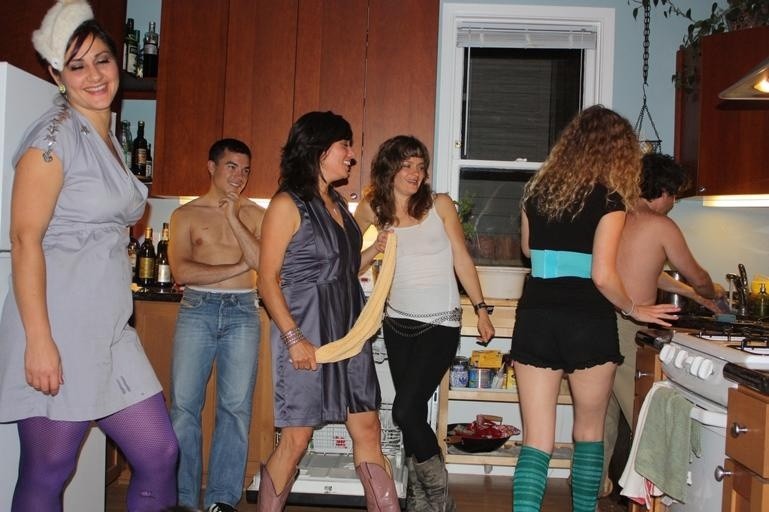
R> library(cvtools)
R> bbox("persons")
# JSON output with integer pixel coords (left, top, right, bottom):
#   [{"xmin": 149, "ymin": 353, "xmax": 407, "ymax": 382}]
[
  {"xmin": 166, "ymin": 137, "xmax": 266, "ymax": 511},
  {"xmin": 505, "ymin": 103, "xmax": 680, "ymax": 512},
  {"xmin": 566, "ymin": 151, "xmax": 727, "ymax": 512},
  {"xmin": 350, "ymin": 133, "xmax": 496, "ymax": 512},
  {"xmin": 1, "ymin": 1, "xmax": 182, "ymax": 512},
  {"xmin": 257, "ymin": 108, "xmax": 401, "ymax": 512}
]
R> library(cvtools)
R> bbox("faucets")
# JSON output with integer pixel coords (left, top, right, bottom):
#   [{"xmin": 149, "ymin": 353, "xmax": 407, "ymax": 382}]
[{"xmin": 726, "ymin": 263, "xmax": 753, "ymax": 317}]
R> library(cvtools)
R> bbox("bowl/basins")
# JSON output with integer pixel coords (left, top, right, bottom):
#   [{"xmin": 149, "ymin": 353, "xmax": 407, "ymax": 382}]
[
  {"xmin": 715, "ymin": 313, "xmax": 736, "ymax": 322},
  {"xmin": 655, "ymin": 270, "xmax": 698, "ymax": 313},
  {"xmin": 446, "ymin": 422, "xmax": 522, "ymax": 453}
]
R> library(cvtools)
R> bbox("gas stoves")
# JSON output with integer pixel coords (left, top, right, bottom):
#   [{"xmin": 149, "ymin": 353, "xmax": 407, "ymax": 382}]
[{"xmin": 657, "ymin": 321, "xmax": 769, "ymax": 410}]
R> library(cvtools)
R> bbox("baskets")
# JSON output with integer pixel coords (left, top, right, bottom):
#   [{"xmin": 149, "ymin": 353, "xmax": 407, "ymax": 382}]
[{"xmin": 639, "ymin": 139, "xmax": 662, "ymax": 155}]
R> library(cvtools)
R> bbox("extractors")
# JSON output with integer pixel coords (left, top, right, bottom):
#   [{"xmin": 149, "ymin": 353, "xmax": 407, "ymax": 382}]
[{"xmin": 715, "ymin": 65, "xmax": 768, "ymax": 111}]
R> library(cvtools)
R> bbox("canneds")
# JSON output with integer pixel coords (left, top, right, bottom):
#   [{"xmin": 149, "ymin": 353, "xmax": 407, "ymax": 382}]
[{"xmin": 450, "ymin": 357, "xmax": 493, "ymax": 389}]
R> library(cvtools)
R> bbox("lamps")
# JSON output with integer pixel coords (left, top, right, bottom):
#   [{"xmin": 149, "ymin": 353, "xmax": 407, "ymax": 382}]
[
  {"xmin": 716, "ymin": 59, "xmax": 769, "ymax": 101},
  {"xmin": 633, "ymin": 0, "xmax": 662, "ymax": 153}
]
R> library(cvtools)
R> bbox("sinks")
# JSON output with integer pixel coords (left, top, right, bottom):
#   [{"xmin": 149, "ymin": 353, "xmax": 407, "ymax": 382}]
[{"xmin": 648, "ymin": 317, "xmax": 735, "ymax": 332}]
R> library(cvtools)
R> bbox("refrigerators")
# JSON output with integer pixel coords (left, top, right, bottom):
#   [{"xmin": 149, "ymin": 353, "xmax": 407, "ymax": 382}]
[{"xmin": 0, "ymin": 59, "xmax": 119, "ymax": 512}]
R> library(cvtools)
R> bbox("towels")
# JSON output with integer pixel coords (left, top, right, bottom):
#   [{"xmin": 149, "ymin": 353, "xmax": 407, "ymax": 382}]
[{"xmin": 617, "ymin": 382, "xmax": 701, "ymax": 512}]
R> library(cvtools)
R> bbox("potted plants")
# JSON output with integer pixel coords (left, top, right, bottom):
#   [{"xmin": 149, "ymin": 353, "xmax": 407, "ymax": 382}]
[{"xmin": 627, "ymin": 0, "xmax": 769, "ymax": 96}]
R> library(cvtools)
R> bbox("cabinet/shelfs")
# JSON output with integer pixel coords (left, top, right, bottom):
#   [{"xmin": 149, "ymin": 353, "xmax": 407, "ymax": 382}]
[
  {"xmin": 152, "ymin": 0, "xmax": 440, "ymax": 203},
  {"xmin": 0, "ymin": 1, "xmax": 127, "ymax": 112},
  {"xmin": 716, "ymin": 363, "xmax": 769, "ymax": 512},
  {"xmin": 431, "ymin": 297, "xmax": 575, "ymax": 467},
  {"xmin": 627, "ymin": 329, "xmax": 670, "ymax": 512},
  {"xmin": 116, "ymin": 77, "xmax": 157, "ymax": 199},
  {"xmin": 117, "ymin": 300, "xmax": 277, "ymax": 490},
  {"xmin": 673, "ymin": 27, "xmax": 769, "ymax": 197}
]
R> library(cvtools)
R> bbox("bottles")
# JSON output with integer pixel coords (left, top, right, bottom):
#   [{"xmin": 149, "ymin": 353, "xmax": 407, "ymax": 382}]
[
  {"xmin": 118, "ymin": 117, "xmax": 153, "ymax": 180},
  {"xmin": 449, "ymin": 354, "xmax": 469, "ymax": 389},
  {"xmin": 156, "ymin": 222, "xmax": 173, "ymax": 292},
  {"xmin": 127, "ymin": 228, "xmax": 139, "ymax": 285},
  {"xmin": 728, "ymin": 260, "xmax": 768, "ymax": 322},
  {"xmin": 139, "ymin": 225, "xmax": 154, "ymax": 290},
  {"xmin": 122, "ymin": 17, "xmax": 157, "ymax": 80}
]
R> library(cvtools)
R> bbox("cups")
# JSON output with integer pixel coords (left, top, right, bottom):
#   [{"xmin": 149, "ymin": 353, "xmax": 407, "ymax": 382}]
[{"xmin": 475, "ymin": 237, "xmax": 522, "ymax": 260}]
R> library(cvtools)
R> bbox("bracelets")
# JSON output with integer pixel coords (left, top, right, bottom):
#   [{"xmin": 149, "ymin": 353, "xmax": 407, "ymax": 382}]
[
  {"xmin": 473, "ymin": 302, "xmax": 494, "ymax": 316},
  {"xmin": 621, "ymin": 297, "xmax": 636, "ymax": 316},
  {"xmin": 279, "ymin": 325, "xmax": 306, "ymax": 349}
]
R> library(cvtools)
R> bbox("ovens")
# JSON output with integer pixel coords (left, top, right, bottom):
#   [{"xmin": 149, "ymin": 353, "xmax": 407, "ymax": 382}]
[{"xmin": 649, "ymin": 379, "xmax": 729, "ymax": 511}]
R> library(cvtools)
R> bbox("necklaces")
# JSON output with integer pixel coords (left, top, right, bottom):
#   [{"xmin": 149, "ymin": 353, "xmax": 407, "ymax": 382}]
[{"xmin": 325, "ymin": 201, "xmax": 341, "ymax": 217}]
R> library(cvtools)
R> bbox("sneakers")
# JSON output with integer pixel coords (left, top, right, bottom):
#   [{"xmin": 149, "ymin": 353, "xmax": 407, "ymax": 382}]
[{"xmin": 207, "ymin": 503, "xmax": 233, "ymax": 512}]
